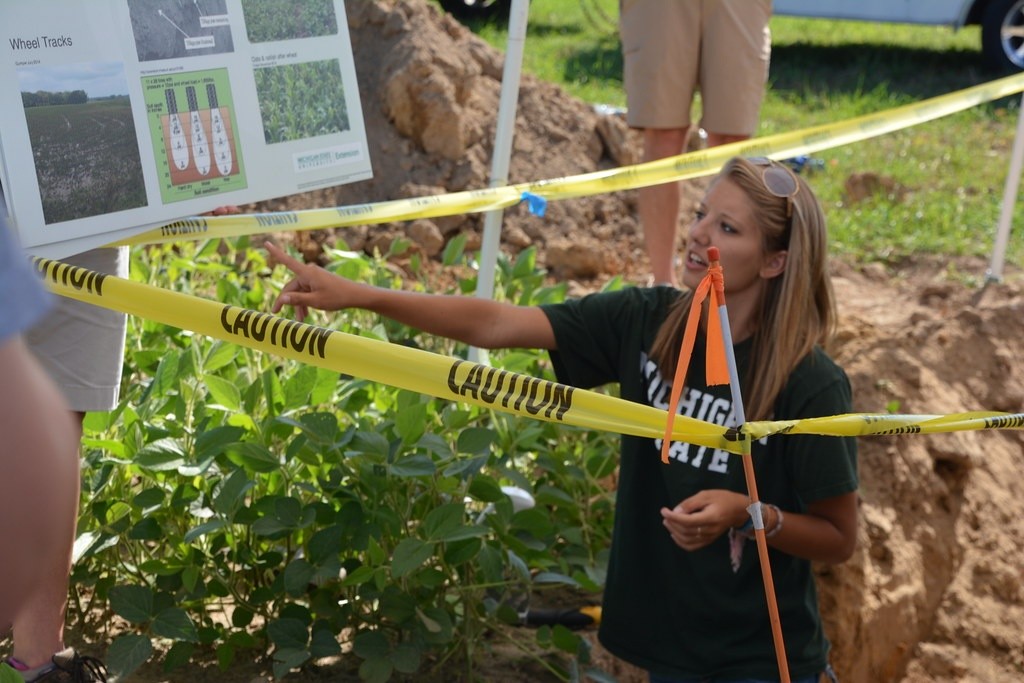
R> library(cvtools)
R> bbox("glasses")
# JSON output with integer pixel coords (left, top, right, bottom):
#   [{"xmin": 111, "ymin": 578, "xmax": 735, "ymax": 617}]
[{"xmin": 747, "ymin": 156, "xmax": 799, "ymax": 217}]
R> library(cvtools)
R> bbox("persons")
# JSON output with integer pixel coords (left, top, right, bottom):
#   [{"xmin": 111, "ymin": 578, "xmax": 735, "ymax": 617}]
[
  {"xmin": 0, "ymin": 198, "xmax": 130, "ymax": 683},
  {"xmin": 619, "ymin": 4, "xmax": 770, "ymax": 289},
  {"xmin": 259, "ymin": 154, "xmax": 857, "ymax": 682}
]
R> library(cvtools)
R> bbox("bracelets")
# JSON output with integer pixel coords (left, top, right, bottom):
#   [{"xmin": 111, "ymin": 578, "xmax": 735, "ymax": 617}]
[{"xmin": 740, "ymin": 503, "xmax": 784, "ymax": 541}]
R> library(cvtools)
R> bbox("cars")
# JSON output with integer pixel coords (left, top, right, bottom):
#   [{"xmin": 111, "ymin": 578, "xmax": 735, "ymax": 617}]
[{"xmin": 429, "ymin": 0, "xmax": 1024, "ymax": 79}]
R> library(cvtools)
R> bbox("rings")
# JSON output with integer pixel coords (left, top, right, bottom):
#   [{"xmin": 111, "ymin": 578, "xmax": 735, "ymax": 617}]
[{"xmin": 697, "ymin": 527, "xmax": 701, "ymax": 536}]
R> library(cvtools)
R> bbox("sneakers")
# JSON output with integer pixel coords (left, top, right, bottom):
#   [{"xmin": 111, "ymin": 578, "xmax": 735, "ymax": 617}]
[{"xmin": 0, "ymin": 646, "xmax": 109, "ymax": 683}]
